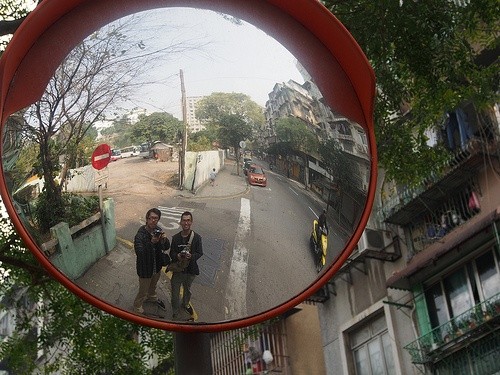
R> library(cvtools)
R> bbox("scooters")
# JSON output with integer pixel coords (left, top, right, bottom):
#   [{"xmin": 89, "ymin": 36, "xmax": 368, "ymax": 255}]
[{"xmin": 310, "ymin": 221, "xmax": 327, "ymax": 271}]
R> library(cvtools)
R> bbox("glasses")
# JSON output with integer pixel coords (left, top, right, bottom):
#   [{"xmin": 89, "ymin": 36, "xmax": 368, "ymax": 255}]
[
  {"xmin": 149, "ymin": 216, "xmax": 159, "ymax": 221},
  {"xmin": 182, "ymin": 219, "xmax": 192, "ymax": 223}
]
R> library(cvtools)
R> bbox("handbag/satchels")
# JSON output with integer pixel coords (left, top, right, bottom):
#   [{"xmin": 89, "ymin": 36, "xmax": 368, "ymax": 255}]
[
  {"xmin": 167, "ymin": 244, "xmax": 192, "ymax": 272},
  {"xmin": 161, "ymin": 253, "xmax": 170, "ymax": 266}
]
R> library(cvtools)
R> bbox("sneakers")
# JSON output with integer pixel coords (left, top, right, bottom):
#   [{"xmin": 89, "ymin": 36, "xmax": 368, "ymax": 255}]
[{"xmin": 184, "ymin": 304, "xmax": 193, "ymax": 315}]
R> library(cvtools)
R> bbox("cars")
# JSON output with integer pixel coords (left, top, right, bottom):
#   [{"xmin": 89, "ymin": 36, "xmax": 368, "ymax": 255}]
[{"xmin": 242, "ymin": 158, "xmax": 267, "ymax": 187}]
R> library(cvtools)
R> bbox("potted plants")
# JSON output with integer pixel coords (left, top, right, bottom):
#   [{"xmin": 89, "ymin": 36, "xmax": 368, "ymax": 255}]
[{"xmin": 423, "ymin": 299, "xmax": 500, "ymax": 353}]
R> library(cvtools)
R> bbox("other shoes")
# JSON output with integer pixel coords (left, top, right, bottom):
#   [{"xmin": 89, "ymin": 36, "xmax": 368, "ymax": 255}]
[{"xmin": 137, "ymin": 305, "xmax": 144, "ymax": 313}]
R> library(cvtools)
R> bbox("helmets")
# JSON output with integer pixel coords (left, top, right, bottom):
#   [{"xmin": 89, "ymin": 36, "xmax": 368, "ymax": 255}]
[{"xmin": 323, "ymin": 210, "xmax": 327, "ymax": 214}]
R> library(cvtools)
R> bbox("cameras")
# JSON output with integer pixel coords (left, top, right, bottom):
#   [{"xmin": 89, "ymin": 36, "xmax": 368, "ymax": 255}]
[
  {"xmin": 154, "ymin": 230, "xmax": 163, "ymax": 238},
  {"xmin": 179, "ymin": 251, "xmax": 192, "ymax": 259}
]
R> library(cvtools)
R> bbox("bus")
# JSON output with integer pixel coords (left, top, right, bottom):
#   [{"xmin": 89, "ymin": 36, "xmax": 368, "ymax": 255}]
[{"xmin": 110, "ymin": 146, "xmax": 141, "ymax": 162}]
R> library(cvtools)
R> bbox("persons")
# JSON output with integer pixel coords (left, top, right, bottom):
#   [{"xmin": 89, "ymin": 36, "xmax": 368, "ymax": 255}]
[
  {"xmin": 316, "ymin": 210, "xmax": 328, "ymax": 247},
  {"xmin": 209, "ymin": 168, "xmax": 217, "ymax": 184},
  {"xmin": 165, "ymin": 211, "xmax": 203, "ymax": 318},
  {"xmin": 133, "ymin": 208, "xmax": 170, "ymax": 312}
]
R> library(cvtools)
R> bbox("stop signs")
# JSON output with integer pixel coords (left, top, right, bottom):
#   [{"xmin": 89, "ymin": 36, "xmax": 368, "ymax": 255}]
[{"xmin": 91, "ymin": 144, "xmax": 112, "ymax": 171}]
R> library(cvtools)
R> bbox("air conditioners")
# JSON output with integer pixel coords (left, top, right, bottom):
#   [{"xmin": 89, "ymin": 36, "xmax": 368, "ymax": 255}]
[{"xmin": 357, "ymin": 228, "xmax": 396, "ymax": 262}]
